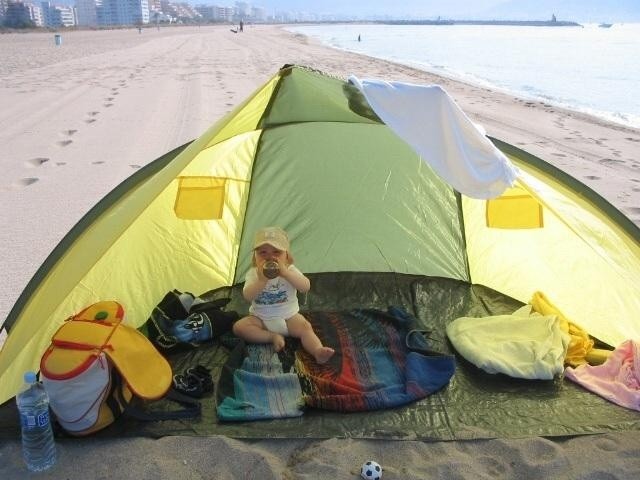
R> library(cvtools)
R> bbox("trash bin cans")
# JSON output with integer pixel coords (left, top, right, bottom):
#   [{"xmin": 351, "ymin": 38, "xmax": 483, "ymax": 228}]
[{"xmin": 55, "ymin": 35, "xmax": 61, "ymax": 45}]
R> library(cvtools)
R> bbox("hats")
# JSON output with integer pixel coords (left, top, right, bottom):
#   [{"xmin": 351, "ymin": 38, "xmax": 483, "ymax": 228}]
[{"xmin": 252, "ymin": 227, "xmax": 289, "ymax": 253}]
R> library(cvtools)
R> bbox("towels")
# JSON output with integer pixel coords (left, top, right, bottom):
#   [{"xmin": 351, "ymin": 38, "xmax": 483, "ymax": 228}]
[
  {"xmin": 343, "ymin": 71, "xmax": 519, "ymax": 201},
  {"xmin": 445, "ymin": 305, "xmax": 570, "ymax": 382}
]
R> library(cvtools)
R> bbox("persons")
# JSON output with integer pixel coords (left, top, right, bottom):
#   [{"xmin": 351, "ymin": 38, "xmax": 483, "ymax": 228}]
[{"xmin": 232, "ymin": 225, "xmax": 335, "ymax": 365}]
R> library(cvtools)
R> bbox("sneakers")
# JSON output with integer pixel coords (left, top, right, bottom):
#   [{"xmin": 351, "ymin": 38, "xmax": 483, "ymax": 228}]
[
  {"xmin": 184, "ymin": 365, "xmax": 214, "ymax": 393},
  {"xmin": 172, "ymin": 374, "xmax": 203, "ymax": 399}
]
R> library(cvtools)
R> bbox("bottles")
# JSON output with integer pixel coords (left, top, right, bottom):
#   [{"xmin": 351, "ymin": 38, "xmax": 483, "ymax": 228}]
[
  {"xmin": 261, "ymin": 261, "xmax": 279, "ymax": 279},
  {"xmin": 15, "ymin": 371, "xmax": 57, "ymax": 471}
]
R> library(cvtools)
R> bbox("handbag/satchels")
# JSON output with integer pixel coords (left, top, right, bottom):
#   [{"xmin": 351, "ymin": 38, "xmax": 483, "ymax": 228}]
[
  {"xmin": 35, "ymin": 300, "xmax": 202, "ymax": 440},
  {"xmin": 146, "ymin": 290, "xmax": 239, "ymax": 357}
]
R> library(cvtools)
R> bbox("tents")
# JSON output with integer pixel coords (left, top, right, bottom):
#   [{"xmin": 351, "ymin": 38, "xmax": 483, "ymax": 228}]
[{"xmin": 0, "ymin": 62, "xmax": 640, "ymax": 449}]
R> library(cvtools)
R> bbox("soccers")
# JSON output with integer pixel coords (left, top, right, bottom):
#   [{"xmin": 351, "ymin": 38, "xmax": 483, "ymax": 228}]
[{"xmin": 361, "ymin": 461, "xmax": 382, "ymax": 480}]
